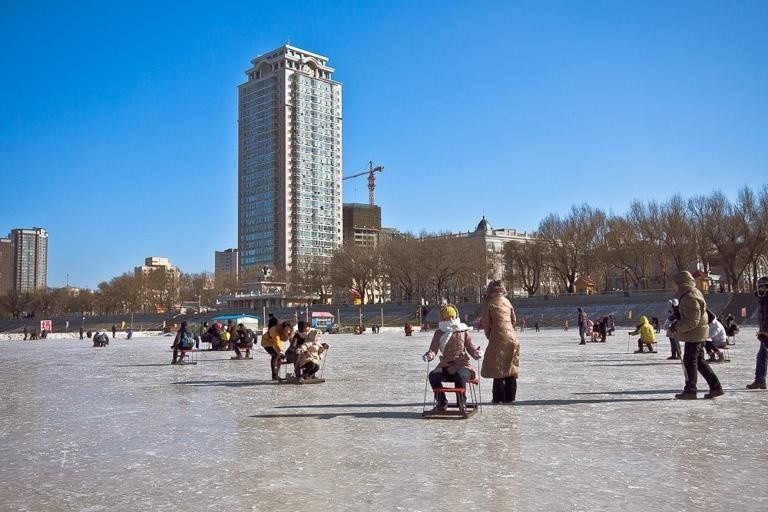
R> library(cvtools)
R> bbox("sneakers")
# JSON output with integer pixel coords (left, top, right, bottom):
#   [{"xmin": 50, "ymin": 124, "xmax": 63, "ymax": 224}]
[
  {"xmin": 675, "ymin": 391, "xmax": 697, "ymax": 401},
  {"xmin": 433, "ymin": 397, "xmax": 515, "ymax": 412},
  {"xmin": 171, "ymin": 346, "xmax": 321, "ymax": 381},
  {"xmin": 704, "ymin": 387, "xmax": 724, "ymax": 399},
  {"xmin": 746, "ymin": 380, "xmax": 767, "ymax": 390}
]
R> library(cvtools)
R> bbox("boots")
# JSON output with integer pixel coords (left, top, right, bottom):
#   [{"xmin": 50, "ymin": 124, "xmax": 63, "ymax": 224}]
[
  {"xmin": 705, "ymin": 353, "xmax": 724, "ymax": 362},
  {"xmin": 579, "ymin": 333, "xmax": 682, "ymax": 360}
]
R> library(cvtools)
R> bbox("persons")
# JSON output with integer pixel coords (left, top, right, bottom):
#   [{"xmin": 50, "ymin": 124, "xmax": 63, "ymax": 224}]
[
  {"xmin": 23, "ymin": 326, "xmax": 47, "ymax": 340},
  {"xmin": 534, "ymin": 321, "xmax": 540, "ymax": 332},
  {"xmin": 404, "ymin": 323, "xmax": 412, "ymax": 336},
  {"xmin": 576, "ymin": 270, "xmax": 739, "ymax": 400},
  {"xmin": 78, "ymin": 323, "xmax": 133, "ymax": 347},
  {"xmin": 423, "ymin": 304, "xmax": 481, "ymax": 415},
  {"xmin": 520, "ymin": 317, "xmax": 526, "ymax": 332},
  {"xmin": 563, "ymin": 319, "xmax": 569, "ymax": 330},
  {"xmin": 354, "ymin": 323, "xmax": 379, "ymax": 334},
  {"xmin": 746, "ymin": 276, "xmax": 768, "ymax": 390},
  {"xmin": 170, "ymin": 312, "xmax": 328, "ymax": 384},
  {"xmin": 480, "ymin": 278, "xmax": 520, "ymax": 403}
]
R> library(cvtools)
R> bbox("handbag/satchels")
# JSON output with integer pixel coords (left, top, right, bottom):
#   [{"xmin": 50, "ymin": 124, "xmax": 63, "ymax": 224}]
[{"xmin": 674, "ymin": 306, "xmax": 716, "ymax": 325}]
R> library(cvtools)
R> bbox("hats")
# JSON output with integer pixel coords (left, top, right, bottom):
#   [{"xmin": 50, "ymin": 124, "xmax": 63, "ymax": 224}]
[{"xmin": 441, "ymin": 305, "xmax": 457, "ymax": 319}]
